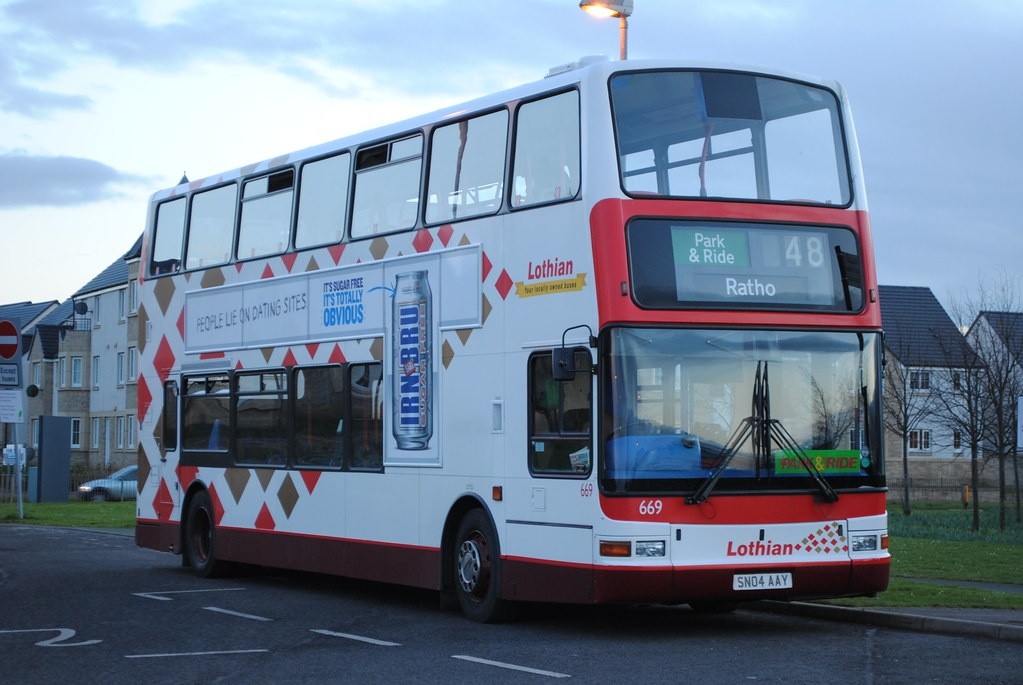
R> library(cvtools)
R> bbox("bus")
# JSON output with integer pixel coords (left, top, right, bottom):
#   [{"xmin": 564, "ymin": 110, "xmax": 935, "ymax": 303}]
[{"xmin": 133, "ymin": 53, "xmax": 894, "ymax": 625}]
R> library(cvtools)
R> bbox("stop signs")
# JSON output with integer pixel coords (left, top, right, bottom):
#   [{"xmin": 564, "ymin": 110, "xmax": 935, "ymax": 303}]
[{"xmin": 0, "ymin": 316, "xmax": 19, "ymax": 360}]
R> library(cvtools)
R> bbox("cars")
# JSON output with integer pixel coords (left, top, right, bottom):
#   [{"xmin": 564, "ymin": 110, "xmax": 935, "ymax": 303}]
[{"xmin": 77, "ymin": 463, "xmax": 139, "ymax": 503}]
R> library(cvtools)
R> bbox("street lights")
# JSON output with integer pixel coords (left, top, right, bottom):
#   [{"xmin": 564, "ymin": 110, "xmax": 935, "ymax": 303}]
[{"xmin": 578, "ymin": 0, "xmax": 636, "ymax": 59}]
